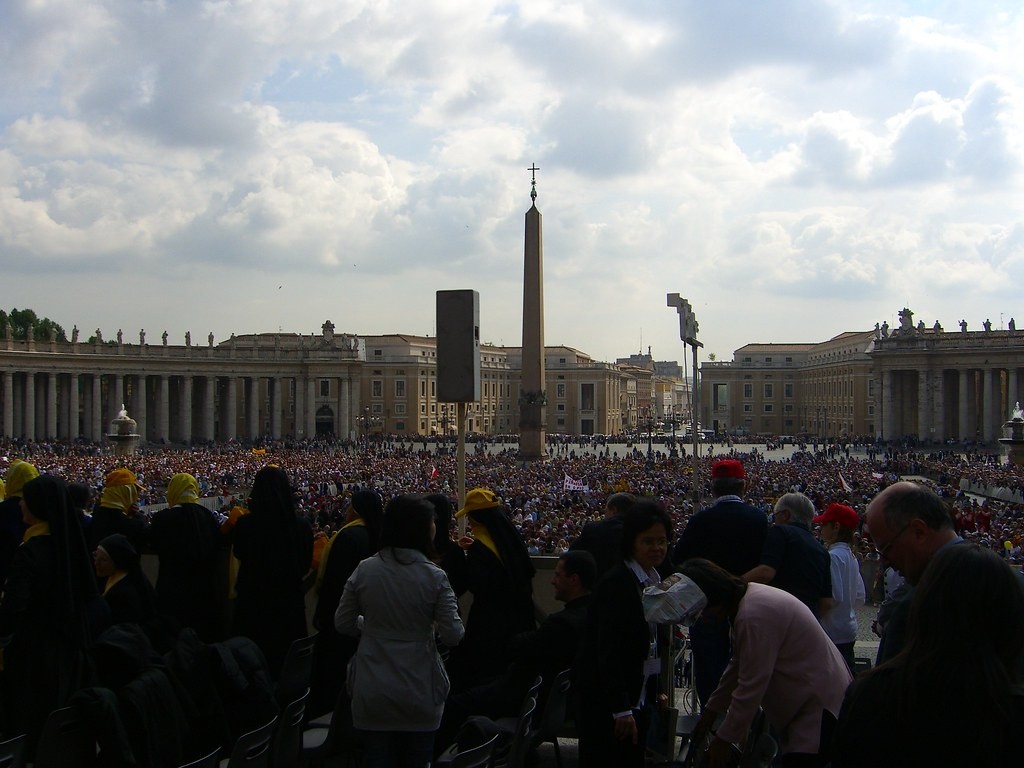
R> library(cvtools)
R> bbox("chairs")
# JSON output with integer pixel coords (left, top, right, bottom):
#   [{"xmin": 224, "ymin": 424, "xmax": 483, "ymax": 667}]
[
  {"xmin": 283, "ymin": 631, "xmax": 320, "ymax": 683},
  {"xmin": 178, "ymin": 745, "xmax": 222, "ymax": 768},
  {"xmin": 448, "ymin": 734, "xmax": 500, "ymax": 768},
  {"xmin": 0, "ymin": 733, "xmax": 29, "ymax": 768},
  {"xmin": 492, "ymin": 668, "xmax": 572, "ymax": 768},
  {"xmin": 220, "ymin": 715, "xmax": 281, "ymax": 768},
  {"xmin": 34, "ymin": 705, "xmax": 72, "ymax": 768},
  {"xmin": 747, "ymin": 733, "xmax": 779, "ymax": 768}
]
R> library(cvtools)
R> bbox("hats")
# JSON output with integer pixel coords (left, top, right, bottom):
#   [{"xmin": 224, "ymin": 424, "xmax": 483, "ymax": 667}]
[
  {"xmin": 712, "ymin": 459, "xmax": 748, "ymax": 479},
  {"xmin": 454, "ymin": 487, "xmax": 501, "ymax": 518},
  {"xmin": 811, "ymin": 504, "xmax": 859, "ymax": 529}
]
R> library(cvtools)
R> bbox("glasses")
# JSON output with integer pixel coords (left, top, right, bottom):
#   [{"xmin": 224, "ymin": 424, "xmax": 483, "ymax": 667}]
[
  {"xmin": 92, "ymin": 551, "xmax": 107, "ymax": 561},
  {"xmin": 772, "ymin": 511, "xmax": 782, "ymax": 522},
  {"xmin": 874, "ymin": 523, "xmax": 911, "ymax": 558},
  {"xmin": 640, "ymin": 536, "xmax": 667, "ymax": 544}
]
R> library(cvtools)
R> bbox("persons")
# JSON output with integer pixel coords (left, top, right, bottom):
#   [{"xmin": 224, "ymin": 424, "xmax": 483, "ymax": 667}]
[
  {"xmin": 185, "ymin": 330, "xmax": 191, "ymax": 346},
  {"xmin": 342, "ymin": 333, "xmax": 348, "ymax": 346},
  {"xmin": 140, "ymin": 329, "xmax": 146, "ymax": 344},
  {"xmin": 297, "ymin": 332, "xmax": 304, "ymax": 346},
  {"xmin": 354, "ymin": 334, "xmax": 359, "ymax": 349},
  {"xmin": 310, "ymin": 332, "xmax": 316, "ymax": 346},
  {"xmin": 5, "ymin": 322, "xmax": 123, "ymax": 344},
  {"xmin": 230, "ymin": 333, "xmax": 236, "ymax": 346},
  {"xmin": 917, "ymin": 320, "xmax": 925, "ymax": 335},
  {"xmin": 983, "ymin": 319, "xmax": 992, "ymax": 332},
  {"xmin": 881, "ymin": 321, "xmax": 889, "ymax": 337},
  {"xmin": 933, "ymin": 320, "xmax": 941, "ymax": 336},
  {"xmin": 254, "ymin": 333, "xmax": 258, "ymax": 345},
  {"xmin": 959, "ymin": 319, "xmax": 967, "ymax": 335},
  {"xmin": 275, "ymin": 333, "xmax": 281, "ymax": 346},
  {"xmin": 874, "ymin": 323, "xmax": 880, "ymax": 339},
  {"xmin": 0, "ymin": 425, "xmax": 1024, "ymax": 768},
  {"xmin": 1008, "ymin": 318, "xmax": 1015, "ymax": 331},
  {"xmin": 208, "ymin": 332, "xmax": 214, "ymax": 347},
  {"xmin": 162, "ymin": 330, "xmax": 168, "ymax": 345}
]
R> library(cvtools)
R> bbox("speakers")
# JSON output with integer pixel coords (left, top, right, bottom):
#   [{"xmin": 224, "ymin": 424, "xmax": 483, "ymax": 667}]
[{"xmin": 437, "ymin": 290, "xmax": 481, "ymax": 402}]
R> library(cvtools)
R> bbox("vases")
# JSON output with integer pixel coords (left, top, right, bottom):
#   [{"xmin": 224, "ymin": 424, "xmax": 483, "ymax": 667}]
[{"xmin": 24, "ymin": 762, "xmax": 34, "ymax": 768}]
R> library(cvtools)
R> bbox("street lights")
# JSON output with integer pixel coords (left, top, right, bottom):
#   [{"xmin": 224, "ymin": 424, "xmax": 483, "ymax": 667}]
[
  {"xmin": 436, "ymin": 412, "xmax": 455, "ymax": 447},
  {"xmin": 641, "ymin": 403, "xmax": 657, "ymax": 452},
  {"xmin": 355, "ymin": 405, "xmax": 380, "ymax": 468}
]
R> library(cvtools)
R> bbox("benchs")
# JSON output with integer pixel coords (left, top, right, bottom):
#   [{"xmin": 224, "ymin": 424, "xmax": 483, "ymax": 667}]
[{"xmin": 273, "ymin": 687, "xmax": 312, "ymax": 768}]
